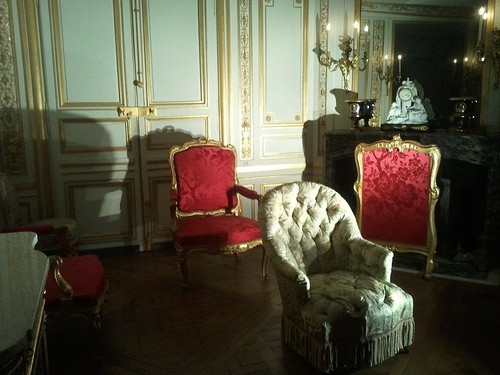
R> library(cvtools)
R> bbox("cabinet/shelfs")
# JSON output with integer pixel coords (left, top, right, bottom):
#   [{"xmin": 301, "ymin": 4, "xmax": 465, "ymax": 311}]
[{"xmin": 0, "ymin": 232, "xmax": 50, "ymax": 375}]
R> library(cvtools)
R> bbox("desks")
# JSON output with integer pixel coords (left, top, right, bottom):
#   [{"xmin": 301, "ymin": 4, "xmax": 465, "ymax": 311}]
[{"xmin": 323, "ymin": 127, "xmax": 500, "ymax": 271}]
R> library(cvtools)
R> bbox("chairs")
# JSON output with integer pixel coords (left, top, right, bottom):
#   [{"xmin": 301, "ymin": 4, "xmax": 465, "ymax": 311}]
[
  {"xmin": 0, "ymin": 174, "xmax": 79, "ymax": 257},
  {"xmin": 169, "ymin": 137, "xmax": 272, "ymax": 291},
  {"xmin": 0, "ymin": 223, "xmax": 110, "ymax": 371},
  {"xmin": 258, "ymin": 182, "xmax": 416, "ymax": 374},
  {"xmin": 354, "ymin": 134, "xmax": 441, "ymax": 280}
]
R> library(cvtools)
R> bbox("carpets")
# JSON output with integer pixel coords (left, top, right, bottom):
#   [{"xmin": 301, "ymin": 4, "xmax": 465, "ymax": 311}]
[{"xmin": 391, "ymin": 247, "xmax": 500, "ymax": 285}]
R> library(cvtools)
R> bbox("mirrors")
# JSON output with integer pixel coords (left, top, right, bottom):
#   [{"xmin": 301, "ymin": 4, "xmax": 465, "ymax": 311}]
[{"xmin": 352, "ymin": 0, "xmax": 496, "ymax": 127}]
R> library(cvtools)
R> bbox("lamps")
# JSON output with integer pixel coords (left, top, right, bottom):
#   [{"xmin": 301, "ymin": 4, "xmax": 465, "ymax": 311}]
[
  {"xmin": 383, "ymin": 55, "xmax": 402, "ymax": 96},
  {"xmin": 325, "ymin": 19, "xmax": 369, "ymax": 92},
  {"xmin": 473, "ymin": 3, "xmax": 500, "ymax": 90},
  {"xmin": 453, "ymin": 57, "xmax": 471, "ymax": 96}
]
